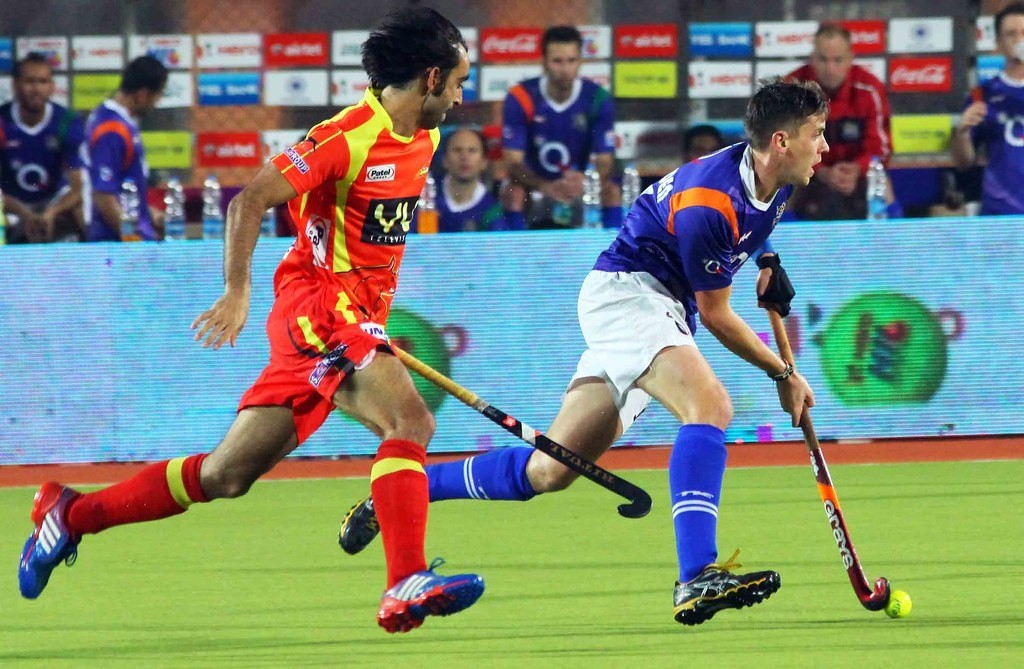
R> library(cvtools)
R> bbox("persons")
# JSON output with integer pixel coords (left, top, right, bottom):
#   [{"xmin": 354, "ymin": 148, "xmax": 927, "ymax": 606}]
[
  {"xmin": 683, "ymin": 124, "xmax": 722, "ymax": 165},
  {"xmin": 954, "ymin": 0, "xmax": 1024, "ymax": 215},
  {"xmin": 0, "ymin": 52, "xmax": 88, "ymax": 243},
  {"xmin": 17, "ymin": 9, "xmax": 486, "ymax": 634},
  {"xmin": 85, "ymin": 56, "xmax": 169, "ymax": 241},
  {"xmin": 338, "ymin": 75, "xmax": 831, "ymax": 626},
  {"xmin": 780, "ymin": 26, "xmax": 895, "ymax": 222},
  {"xmin": 498, "ymin": 25, "xmax": 622, "ymax": 231},
  {"xmin": 409, "ymin": 127, "xmax": 511, "ymax": 233}
]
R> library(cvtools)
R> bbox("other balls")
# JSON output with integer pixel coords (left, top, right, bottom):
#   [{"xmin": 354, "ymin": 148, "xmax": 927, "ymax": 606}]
[{"xmin": 885, "ymin": 589, "xmax": 911, "ymax": 619}]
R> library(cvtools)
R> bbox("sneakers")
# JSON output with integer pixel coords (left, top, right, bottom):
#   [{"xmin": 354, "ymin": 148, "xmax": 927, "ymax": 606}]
[
  {"xmin": 18, "ymin": 481, "xmax": 82, "ymax": 599},
  {"xmin": 377, "ymin": 557, "xmax": 484, "ymax": 634},
  {"xmin": 673, "ymin": 548, "xmax": 780, "ymax": 625},
  {"xmin": 338, "ymin": 492, "xmax": 380, "ymax": 555}
]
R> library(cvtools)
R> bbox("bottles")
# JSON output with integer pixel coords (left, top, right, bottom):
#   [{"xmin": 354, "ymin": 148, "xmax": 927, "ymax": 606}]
[
  {"xmin": 118, "ymin": 176, "xmax": 140, "ymax": 241},
  {"xmin": 552, "ymin": 163, "xmax": 578, "ymax": 226},
  {"xmin": 417, "ymin": 170, "xmax": 438, "ymax": 234},
  {"xmin": 865, "ymin": 156, "xmax": 888, "ymax": 220},
  {"xmin": 201, "ymin": 175, "xmax": 224, "ymax": 240},
  {"xmin": 261, "ymin": 207, "xmax": 277, "ymax": 239},
  {"xmin": 580, "ymin": 164, "xmax": 602, "ymax": 229},
  {"xmin": 163, "ymin": 175, "xmax": 187, "ymax": 242},
  {"xmin": 620, "ymin": 162, "xmax": 642, "ymax": 228}
]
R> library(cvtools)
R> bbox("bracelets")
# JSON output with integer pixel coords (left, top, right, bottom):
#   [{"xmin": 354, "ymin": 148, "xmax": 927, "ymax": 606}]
[{"xmin": 764, "ymin": 359, "xmax": 793, "ymax": 381}]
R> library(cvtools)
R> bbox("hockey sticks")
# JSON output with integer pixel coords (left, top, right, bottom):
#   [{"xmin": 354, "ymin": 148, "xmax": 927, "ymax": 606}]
[
  {"xmin": 764, "ymin": 305, "xmax": 891, "ymax": 611},
  {"xmin": 387, "ymin": 341, "xmax": 652, "ymax": 519}
]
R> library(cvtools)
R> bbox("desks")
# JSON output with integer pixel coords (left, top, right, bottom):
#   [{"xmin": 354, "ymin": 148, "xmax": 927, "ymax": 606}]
[{"xmin": 0, "ymin": 214, "xmax": 1024, "ymax": 488}]
